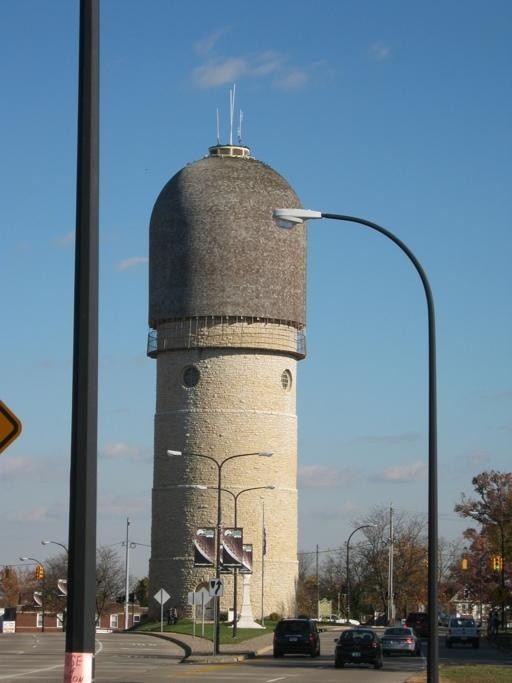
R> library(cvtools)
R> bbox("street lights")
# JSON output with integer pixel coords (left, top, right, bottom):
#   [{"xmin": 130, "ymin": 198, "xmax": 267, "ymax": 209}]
[
  {"xmin": 345, "ymin": 524, "xmax": 378, "ymax": 625},
  {"xmin": 18, "ymin": 556, "xmax": 45, "ymax": 633},
  {"xmin": 468, "ymin": 510, "xmax": 504, "ymax": 630},
  {"xmin": 167, "ymin": 448, "xmax": 277, "ymax": 656}
]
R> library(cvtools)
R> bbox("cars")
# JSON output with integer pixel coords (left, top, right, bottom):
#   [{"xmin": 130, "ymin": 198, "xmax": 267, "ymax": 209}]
[
  {"xmin": 333, "ymin": 629, "xmax": 384, "ymax": 669},
  {"xmin": 270, "ymin": 206, "xmax": 441, "ymax": 682},
  {"xmin": 380, "ymin": 626, "xmax": 422, "ymax": 657}
]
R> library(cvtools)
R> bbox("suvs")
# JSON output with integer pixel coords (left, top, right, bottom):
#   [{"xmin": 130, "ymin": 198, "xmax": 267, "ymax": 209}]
[
  {"xmin": 445, "ymin": 617, "xmax": 482, "ymax": 649},
  {"xmin": 404, "ymin": 613, "xmax": 431, "ymax": 638},
  {"xmin": 272, "ymin": 617, "xmax": 320, "ymax": 658}
]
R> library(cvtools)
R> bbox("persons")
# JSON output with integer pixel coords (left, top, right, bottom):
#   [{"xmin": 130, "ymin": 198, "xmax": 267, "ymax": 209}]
[
  {"xmin": 173, "ymin": 607, "xmax": 178, "ymax": 624},
  {"xmin": 167, "ymin": 607, "xmax": 173, "ymax": 624}
]
